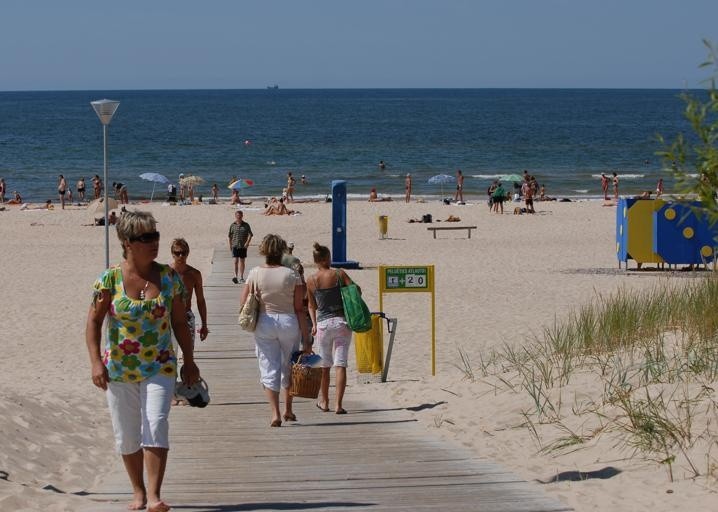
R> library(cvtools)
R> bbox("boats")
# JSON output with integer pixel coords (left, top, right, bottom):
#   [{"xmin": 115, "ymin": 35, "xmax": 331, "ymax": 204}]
[{"xmin": 266, "ymin": 83, "xmax": 280, "ymax": 89}]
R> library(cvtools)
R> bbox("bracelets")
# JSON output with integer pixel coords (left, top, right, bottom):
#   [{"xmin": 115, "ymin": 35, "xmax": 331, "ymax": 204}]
[{"xmin": 203, "ymin": 321, "xmax": 207, "ymax": 327}]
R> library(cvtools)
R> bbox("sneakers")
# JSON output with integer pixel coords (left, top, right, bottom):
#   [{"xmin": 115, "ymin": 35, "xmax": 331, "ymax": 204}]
[{"xmin": 172, "ymin": 382, "xmax": 210, "ymax": 408}]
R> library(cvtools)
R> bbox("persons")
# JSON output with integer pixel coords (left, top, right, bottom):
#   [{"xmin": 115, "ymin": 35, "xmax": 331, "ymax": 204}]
[
  {"xmin": 169, "ymin": 238, "xmax": 208, "ymax": 406},
  {"xmin": 454, "ymin": 170, "xmax": 464, "ymax": 200},
  {"xmin": 86, "ymin": 211, "xmax": 199, "ymax": 512},
  {"xmin": 487, "ymin": 170, "xmax": 547, "ymax": 215},
  {"xmin": 378, "ymin": 161, "xmax": 385, "ymax": 167},
  {"xmin": 239, "ymin": 233, "xmax": 311, "ymax": 426},
  {"xmin": 404, "ymin": 173, "xmax": 411, "ymax": 203},
  {"xmin": 227, "ymin": 211, "xmax": 253, "ymax": 284},
  {"xmin": 306, "ymin": 242, "xmax": 361, "ymax": 414},
  {"xmin": 280, "ymin": 241, "xmax": 299, "ymax": 268},
  {"xmin": 290, "ymin": 262, "xmax": 316, "ymax": 355},
  {"xmin": 601, "ymin": 171, "xmax": 662, "ymax": 199},
  {"xmin": 368, "ymin": 188, "xmax": 394, "ymax": 203}
]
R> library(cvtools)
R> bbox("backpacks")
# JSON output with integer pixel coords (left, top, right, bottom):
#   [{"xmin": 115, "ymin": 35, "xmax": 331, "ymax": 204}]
[{"xmin": 237, "ymin": 267, "xmax": 259, "ymax": 330}]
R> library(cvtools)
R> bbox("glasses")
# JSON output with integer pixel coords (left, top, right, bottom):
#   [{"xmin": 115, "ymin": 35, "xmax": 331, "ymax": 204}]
[
  {"xmin": 172, "ymin": 250, "xmax": 187, "ymax": 256},
  {"xmin": 124, "ymin": 231, "xmax": 160, "ymax": 244}
]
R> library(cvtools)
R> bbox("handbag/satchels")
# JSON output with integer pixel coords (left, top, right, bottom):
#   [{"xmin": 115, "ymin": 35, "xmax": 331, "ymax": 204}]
[{"xmin": 334, "ymin": 267, "xmax": 371, "ymax": 332}]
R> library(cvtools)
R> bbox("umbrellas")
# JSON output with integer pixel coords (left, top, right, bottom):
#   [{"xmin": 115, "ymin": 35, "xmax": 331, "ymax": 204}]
[{"xmin": 428, "ymin": 173, "xmax": 455, "ymax": 199}]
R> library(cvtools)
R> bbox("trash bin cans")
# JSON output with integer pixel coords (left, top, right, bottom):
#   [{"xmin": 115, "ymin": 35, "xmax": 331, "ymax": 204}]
[
  {"xmin": 378, "ymin": 215, "xmax": 388, "ymax": 234},
  {"xmin": 355, "ymin": 313, "xmax": 384, "ymax": 374}
]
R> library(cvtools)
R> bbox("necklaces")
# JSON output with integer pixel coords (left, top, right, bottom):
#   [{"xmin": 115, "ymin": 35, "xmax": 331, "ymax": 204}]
[{"xmin": 140, "ymin": 279, "xmax": 151, "ymax": 300}]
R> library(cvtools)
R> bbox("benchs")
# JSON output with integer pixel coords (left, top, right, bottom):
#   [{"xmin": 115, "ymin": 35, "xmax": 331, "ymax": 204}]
[{"xmin": 426, "ymin": 226, "xmax": 477, "ymax": 239}]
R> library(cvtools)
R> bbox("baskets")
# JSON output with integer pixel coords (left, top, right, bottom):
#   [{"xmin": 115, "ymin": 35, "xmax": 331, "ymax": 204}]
[{"xmin": 290, "ymin": 350, "xmax": 322, "ymax": 398}]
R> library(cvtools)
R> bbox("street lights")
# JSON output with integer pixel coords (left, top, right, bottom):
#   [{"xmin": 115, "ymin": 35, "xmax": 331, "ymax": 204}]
[{"xmin": 88, "ymin": 96, "xmax": 123, "ymax": 270}]
